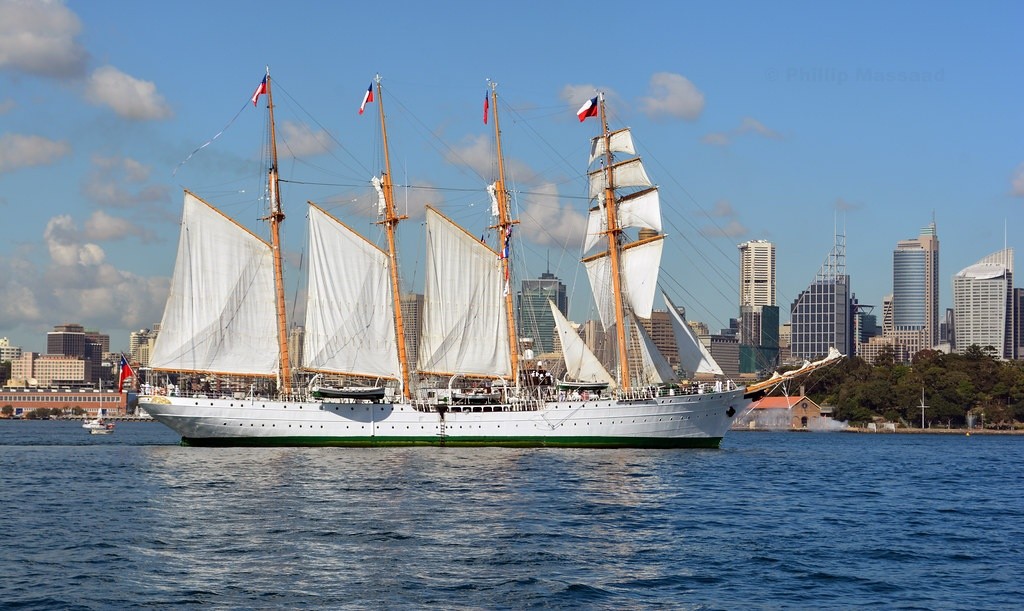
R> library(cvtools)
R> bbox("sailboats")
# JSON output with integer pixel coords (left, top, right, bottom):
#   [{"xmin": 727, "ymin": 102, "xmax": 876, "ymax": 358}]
[{"xmin": 136, "ymin": 65, "xmax": 847, "ymax": 448}]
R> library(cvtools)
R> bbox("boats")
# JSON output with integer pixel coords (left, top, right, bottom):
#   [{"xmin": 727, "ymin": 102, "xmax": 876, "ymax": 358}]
[{"xmin": 82, "ymin": 378, "xmax": 118, "ymax": 435}]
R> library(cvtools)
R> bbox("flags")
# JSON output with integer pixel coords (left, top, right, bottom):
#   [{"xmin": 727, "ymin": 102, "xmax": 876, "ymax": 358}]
[
  {"xmin": 577, "ymin": 96, "xmax": 598, "ymax": 123},
  {"xmin": 119, "ymin": 353, "xmax": 133, "ymax": 395},
  {"xmin": 359, "ymin": 82, "xmax": 374, "ymax": 114},
  {"xmin": 252, "ymin": 74, "xmax": 266, "ymax": 106},
  {"xmin": 483, "ymin": 90, "xmax": 489, "ymax": 124},
  {"xmin": 498, "ymin": 228, "xmax": 511, "ymax": 259}
]
